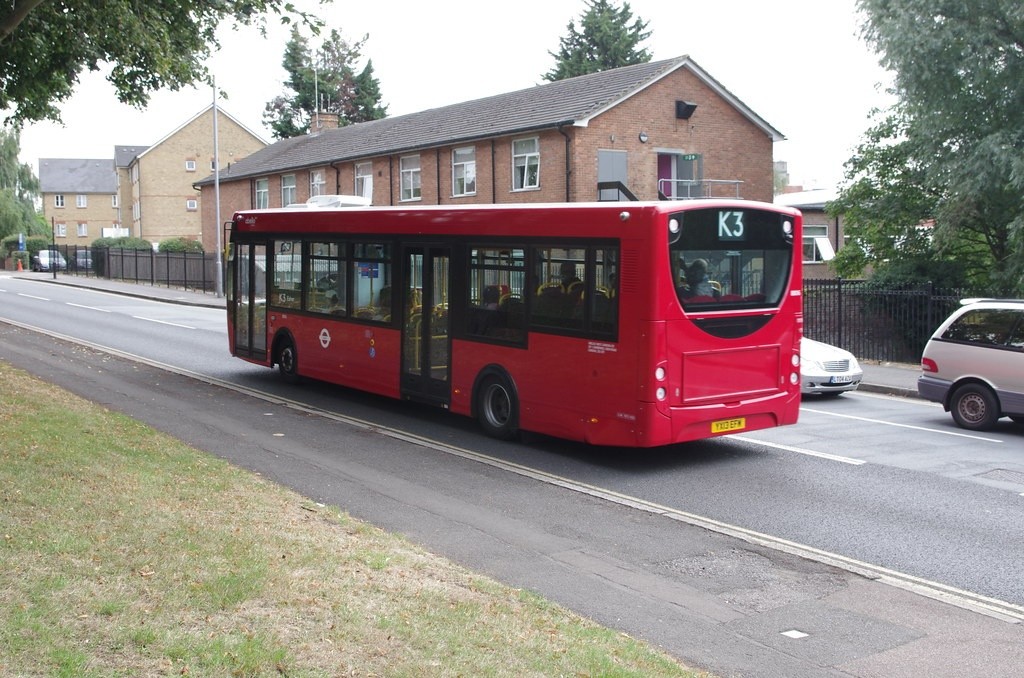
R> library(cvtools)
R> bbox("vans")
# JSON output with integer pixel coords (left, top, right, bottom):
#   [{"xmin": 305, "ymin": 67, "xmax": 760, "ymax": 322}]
[{"xmin": 918, "ymin": 298, "xmax": 1024, "ymax": 431}]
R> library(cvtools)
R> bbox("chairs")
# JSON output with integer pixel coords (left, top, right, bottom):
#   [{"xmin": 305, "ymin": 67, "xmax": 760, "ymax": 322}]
[{"xmin": 241, "ymin": 277, "xmax": 766, "ymax": 368}]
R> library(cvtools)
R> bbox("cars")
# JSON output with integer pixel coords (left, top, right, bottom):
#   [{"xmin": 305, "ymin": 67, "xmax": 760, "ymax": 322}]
[{"xmin": 801, "ymin": 335, "xmax": 862, "ymax": 397}]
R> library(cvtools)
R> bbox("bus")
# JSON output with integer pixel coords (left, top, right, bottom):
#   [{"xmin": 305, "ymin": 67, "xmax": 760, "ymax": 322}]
[{"xmin": 222, "ymin": 195, "xmax": 800, "ymax": 447}]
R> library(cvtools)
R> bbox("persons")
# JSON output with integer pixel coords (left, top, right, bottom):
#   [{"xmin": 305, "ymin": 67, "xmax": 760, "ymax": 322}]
[
  {"xmin": 323, "ymin": 272, "xmax": 338, "ymax": 285},
  {"xmin": 678, "ymin": 252, "xmax": 714, "ymax": 298},
  {"xmin": 478, "ymin": 285, "xmax": 500, "ymax": 309},
  {"xmin": 372, "ymin": 286, "xmax": 393, "ymax": 322},
  {"xmin": 553, "ymin": 261, "xmax": 586, "ymax": 294}
]
